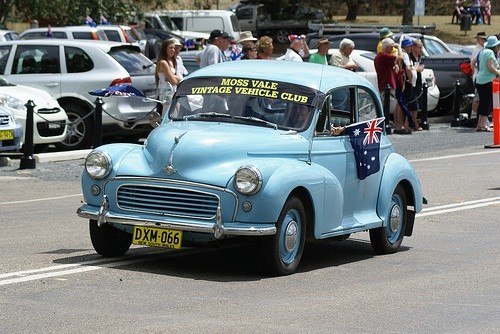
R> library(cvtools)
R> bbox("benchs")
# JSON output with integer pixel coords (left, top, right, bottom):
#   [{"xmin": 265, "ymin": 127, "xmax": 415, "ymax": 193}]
[{"xmin": 265, "ymin": 113, "xmax": 350, "ymax": 126}]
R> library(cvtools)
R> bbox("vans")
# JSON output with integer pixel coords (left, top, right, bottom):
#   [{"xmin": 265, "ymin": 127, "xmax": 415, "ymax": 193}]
[{"xmin": 149, "ymin": 9, "xmax": 241, "ymax": 45}]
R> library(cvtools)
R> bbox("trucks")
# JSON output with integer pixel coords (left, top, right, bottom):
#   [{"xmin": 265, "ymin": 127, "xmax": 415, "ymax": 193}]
[{"xmin": 234, "ymin": 3, "xmax": 308, "ymax": 43}]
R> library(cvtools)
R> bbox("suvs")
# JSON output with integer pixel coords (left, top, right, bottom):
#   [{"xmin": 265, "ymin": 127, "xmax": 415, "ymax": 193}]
[
  {"xmin": 114, "ymin": 12, "xmax": 212, "ymax": 44},
  {"xmin": 0, "ymin": 38, "xmax": 171, "ymax": 150}
]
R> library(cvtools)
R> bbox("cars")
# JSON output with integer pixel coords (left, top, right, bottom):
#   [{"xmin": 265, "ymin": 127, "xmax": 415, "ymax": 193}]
[
  {"xmin": 0, "ymin": 24, "xmax": 187, "ymax": 69},
  {"xmin": 0, "ymin": 74, "xmax": 69, "ymax": 155},
  {"xmin": 275, "ymin": 48, "xmax": 441, "ymax": 120},
  {"xmin": 75, "ymin": 58, "xmax": 427, "ymax": 276},
  {"xmin": 304, "ymin": 29, "xmax": 476, "ymax": 105}
]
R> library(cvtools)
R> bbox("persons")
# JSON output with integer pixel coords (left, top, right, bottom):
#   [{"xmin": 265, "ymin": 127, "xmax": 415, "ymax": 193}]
[
  {"xmin": 330, "ymin": 38, "xmax": 357, "ymax": 116},
  {"xmin": 169, "ymin": 38, "xmax": 189, "ymax": 78},
  {"xmin": 452, "ymin": 0, "xmax": 491, "ymax": 25},
  {"xmin": 470, "ymin": 32, "xmax": 500, "ymax": 132},
  {"xmin": 285, "ymin": 97, "xmax": 344, "ymax": 135},
  {"xmin": 155, "ymin": 40, "xmax": 183, "ymax": 104},
  {"xmin": 196, "ymin": 29, "xmax": 274, "ymax": 70},
  {"xmin": 284, "ymin": 34, "xmax": 309, "ymax": 62},
  {"xmin": 309, "ymin": 38, "xmax": 330, "ymax": 65},
  {"xmin": 374, "ymin": 28, "xmax": 430, "ymax": 135}
]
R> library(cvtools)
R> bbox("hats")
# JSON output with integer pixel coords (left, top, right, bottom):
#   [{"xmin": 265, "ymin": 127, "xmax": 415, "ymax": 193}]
[
  {"xmin": 401, "ymin": 38, "xmax": 413, "ymax": 48},
  {"xmin": 378, "ymin": 27, "xmax": 394, "ymax": 39},
  {"xmin": 474, "ymin": 32, "xmax": 488, "ymax": 39},
  {"xmin": 288, "ymin": 34, "xmax": 303, "ymax": 43},
  {"xmin": 210, "ymin": 30, "xmax": 227, "ymax": 38},
  {"xmin": 170, "ymin": 37, "xmax": 183, "ymax": 46},
  {"xmin": 316, "ymin": 39, "xmax": 333, "ymax": 45},
  {"xmin": 221, "ymin": 31, "xmax": 235, "ymax": 40},
  {"xmin": 236, "ymin": 30, "xmax": 258, "ymax": 44},
  {"xmin": 484, "ymin": 36, "xmax": 500, "ymax": 48}
]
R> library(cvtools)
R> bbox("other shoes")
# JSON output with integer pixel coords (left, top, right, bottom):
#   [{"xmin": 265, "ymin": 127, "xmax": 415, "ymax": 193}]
[
  {"xmin": 384, "ymin": 117, "xmax": 430, "ymax": 134},
  {"xmin": 474, "ymin": 125, "xmax": 494, "ymax": 133},
  {"xmin": 469, "ymin": 118, "xmax": 478, "ymax": 127}
]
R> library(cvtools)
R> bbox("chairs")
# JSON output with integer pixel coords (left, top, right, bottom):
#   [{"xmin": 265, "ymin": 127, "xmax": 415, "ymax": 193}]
[
  {"xmin": 19, "ymin": 55, "xmax": 36, "ymax": 74},
  {"xmin": 38, "ymin": 53, "xmax": 51, "ymax": 73}
]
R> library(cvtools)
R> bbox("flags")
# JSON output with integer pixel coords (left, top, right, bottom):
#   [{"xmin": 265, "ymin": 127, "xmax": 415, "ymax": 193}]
[{"xmin": 344, "ymin": 117, "xmax": 385, "ymax": 180}]
